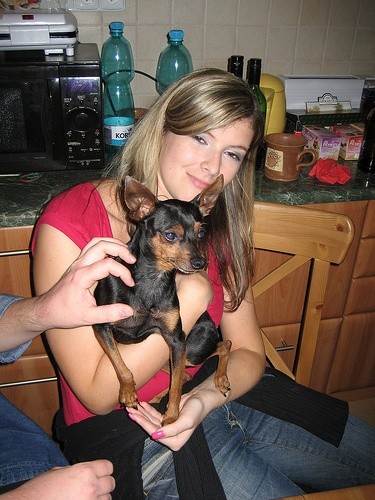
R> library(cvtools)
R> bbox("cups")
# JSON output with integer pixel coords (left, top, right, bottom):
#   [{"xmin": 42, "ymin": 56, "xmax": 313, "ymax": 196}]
[
  {"xmin": 263, "ymin": 132, "xmax": 317, "ymax": 182},
  {"xmin": 115, "ymin": 107, "xmax": 150, "ymax": 122}
]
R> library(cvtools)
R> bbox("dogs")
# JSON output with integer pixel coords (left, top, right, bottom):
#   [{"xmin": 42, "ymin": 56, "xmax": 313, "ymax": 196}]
[{"xmin": 91, "ymin": 173, "xmax": 233, "ymax": 428}]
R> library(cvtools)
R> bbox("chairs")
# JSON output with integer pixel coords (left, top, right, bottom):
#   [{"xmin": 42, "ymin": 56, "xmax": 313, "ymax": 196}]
[{"xmin": 251, "ymin": 201, "xmax": 355, "ymax": 390}]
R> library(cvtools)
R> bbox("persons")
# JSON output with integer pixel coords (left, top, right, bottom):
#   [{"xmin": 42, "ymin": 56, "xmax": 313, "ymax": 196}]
[
  {"xmin": 29, "ymin": 68, "xmax": 375, "ymax": 500},
  {"xmin": 0, "ymin": 234, "xmax": 136, "ymax": 500}
]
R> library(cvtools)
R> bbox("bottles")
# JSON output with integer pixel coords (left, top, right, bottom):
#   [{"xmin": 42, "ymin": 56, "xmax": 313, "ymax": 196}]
[
  {"xmin": 155, "ymin": 30, "xmax": 194, "ymax": 96},
  {"xmin": 229, "ymin": 55, "xmax": 244, "ymax": 78},
  {"xmin": 245, "ymin": 58, "xmax": 267, "ymax": 120},
  {"xmin": 356, "ymin": 102, "xmax": 375, "ymax": 173},
  {"xmin": 100, "ymin": 21, "xmax": 136, "ymax": 155}
]
